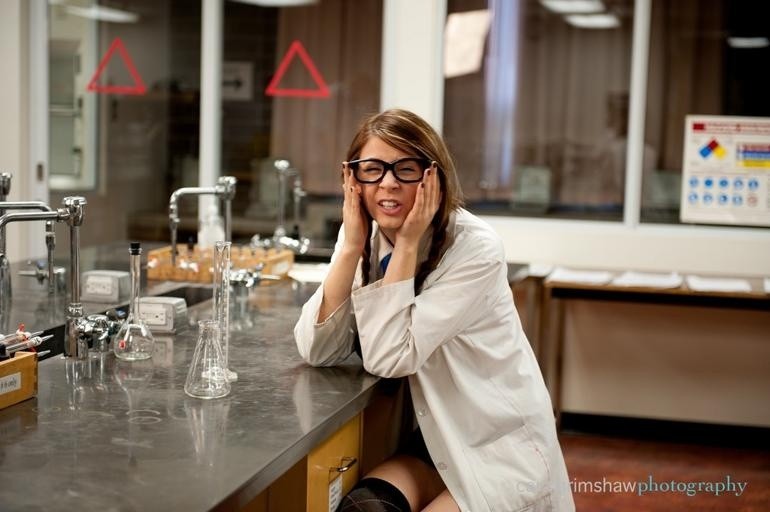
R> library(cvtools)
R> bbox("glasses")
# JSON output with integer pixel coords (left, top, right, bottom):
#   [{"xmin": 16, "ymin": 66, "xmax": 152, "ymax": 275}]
[{"xmin": 347, "ymin": 158, "xmax": 431, "ymax": 183}]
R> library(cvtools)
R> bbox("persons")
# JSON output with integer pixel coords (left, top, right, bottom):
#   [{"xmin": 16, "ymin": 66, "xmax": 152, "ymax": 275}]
[{"xmin": 293, "ymin": 108, "xmax": 577, "ymax": 511}]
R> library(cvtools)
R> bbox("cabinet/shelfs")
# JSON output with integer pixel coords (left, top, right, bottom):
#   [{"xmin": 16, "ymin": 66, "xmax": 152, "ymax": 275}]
[{"xmin": 0, "ymin": 239, "xmax": 770, "ymax": 512}]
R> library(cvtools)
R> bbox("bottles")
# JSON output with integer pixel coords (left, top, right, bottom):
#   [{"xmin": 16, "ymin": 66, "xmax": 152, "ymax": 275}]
[
  {"xmin": 112, "ymin": 242, "xmax": 156, "ymax": 361},
  {"xmin": 212, "ymin": 241, "xmax": 238, "ymax": 384},
  {"xmin": 183, "ymin": 319, "xmax": 232, "ymax": 400}
]
